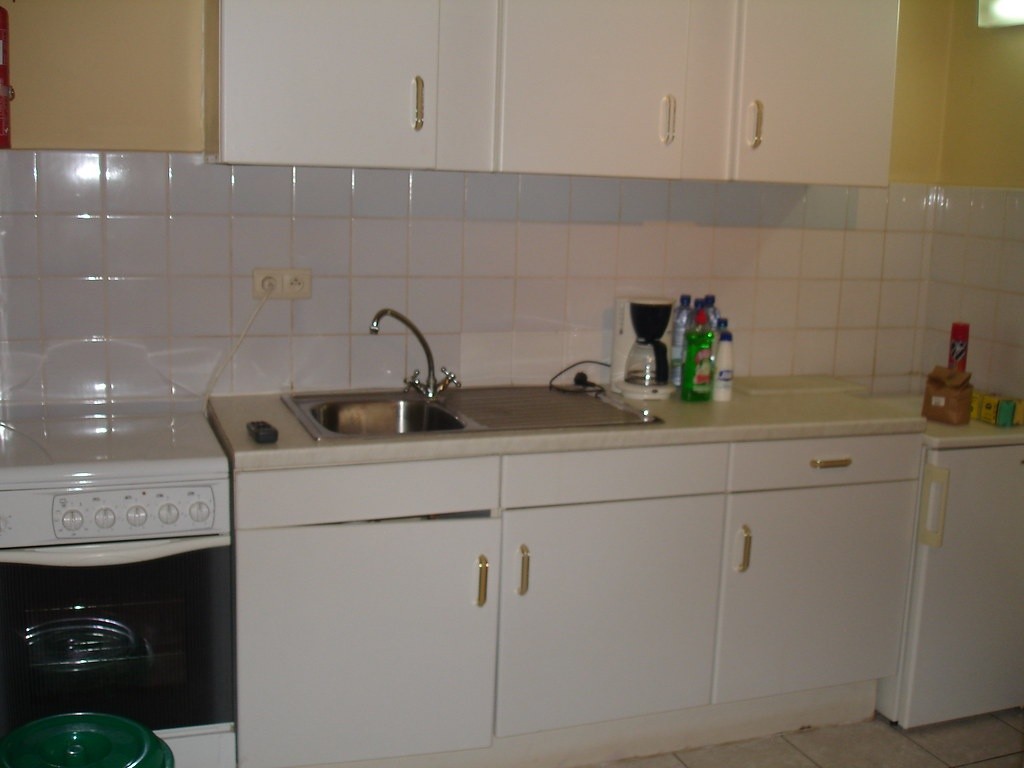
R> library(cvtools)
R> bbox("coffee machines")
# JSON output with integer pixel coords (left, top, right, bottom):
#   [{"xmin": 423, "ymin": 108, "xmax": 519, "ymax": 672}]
[{"xmin": 612, "ymin": 297, "xmax": 672, "ymax": 400}]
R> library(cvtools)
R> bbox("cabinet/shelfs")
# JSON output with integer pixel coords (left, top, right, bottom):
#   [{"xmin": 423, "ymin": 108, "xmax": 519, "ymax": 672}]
[
  {"xmin": 0, "ymin": 0, "xmax": 220, "ymax": 153},
  {"xmin": 209, "ymin": 0, "xmax": 502, "ymax": 175},
  {"xmin": 205, "ymin": 455, "xmax": 501, "ymax": 768},
  {"xmin": 876, "ymin": 443, "xmax": 1024, "ymax": 730},
  {"xmin": 493, "ymin": 434, "xmax": 922, "ymax": 768},
  {"xmin": 497, "ymin": 0, "xmax": 901, "ymax": 189}
]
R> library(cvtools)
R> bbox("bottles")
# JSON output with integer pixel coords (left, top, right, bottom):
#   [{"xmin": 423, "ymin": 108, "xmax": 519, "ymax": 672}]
[
  {"xmin": 672, "ymin": 294, "xmax": 734, "ymax": 402},
  {"xmin": 949, "ymin": 322, "xmax": 969, "ymax": 371}
]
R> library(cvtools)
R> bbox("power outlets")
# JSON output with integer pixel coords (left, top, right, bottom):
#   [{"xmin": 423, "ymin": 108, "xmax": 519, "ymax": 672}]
[{"xmin": 252, "ymin": 267, "xmax": 312, "ymax": 302}]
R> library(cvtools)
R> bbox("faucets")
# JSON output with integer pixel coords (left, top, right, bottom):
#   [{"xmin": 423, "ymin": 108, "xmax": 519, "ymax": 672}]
[{"xmin": 370, "ymin": 309, "xmax": 437, "ymax": 397}]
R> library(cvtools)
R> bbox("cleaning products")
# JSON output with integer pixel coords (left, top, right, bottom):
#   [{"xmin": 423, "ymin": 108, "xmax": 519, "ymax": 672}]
[{"xmin": 682, "ymin": 311, "xmax": 734, "ymax": 404}]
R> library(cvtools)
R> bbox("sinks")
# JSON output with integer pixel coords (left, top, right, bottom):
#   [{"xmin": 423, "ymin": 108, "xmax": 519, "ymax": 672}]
[{"xmin": 279, "ymin": 389, "xmax": 492, "ymax": 447}]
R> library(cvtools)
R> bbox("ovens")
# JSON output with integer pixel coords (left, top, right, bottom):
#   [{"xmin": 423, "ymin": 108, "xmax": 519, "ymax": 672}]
[{"xmin": 0, "ymin": 414, "xmax": 236, "ymax": 768}]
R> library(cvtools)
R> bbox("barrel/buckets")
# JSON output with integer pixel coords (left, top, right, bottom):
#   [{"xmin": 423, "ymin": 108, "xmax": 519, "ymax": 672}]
[{"xmin": 0, "ymin": 713, "xmax": 174, "ymax": 768}]
